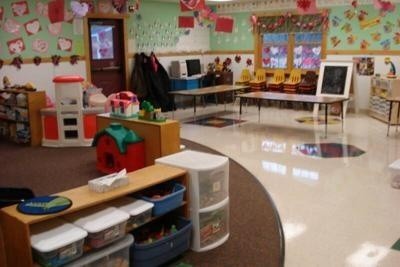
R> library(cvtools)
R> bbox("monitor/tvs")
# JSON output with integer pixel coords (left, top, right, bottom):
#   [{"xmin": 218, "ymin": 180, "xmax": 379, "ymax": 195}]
[{"xmin": 187, "ymin": 59, "xmax": 201, "ymax": 75}]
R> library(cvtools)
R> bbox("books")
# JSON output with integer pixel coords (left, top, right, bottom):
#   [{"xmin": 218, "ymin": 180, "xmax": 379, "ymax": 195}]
[{"xmin": 0, "ymin": 92, "xmax": 29, "ymax": 144}]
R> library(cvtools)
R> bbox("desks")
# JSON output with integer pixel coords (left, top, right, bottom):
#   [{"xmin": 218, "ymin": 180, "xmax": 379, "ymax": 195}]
[
  {"xmin": 0, "ymin": 136, "xmax": 285, "ymax": 267},
  {"xmin": 385, "ymin": 97, "xmax": 400, "ymax": 136},
  {"xmin": 167, "ymin": 83, "xmax": 251, "ymax": 121},
  {"xmin": 235, "ymin": 90, "xmax": 349, "ymax": 138}
]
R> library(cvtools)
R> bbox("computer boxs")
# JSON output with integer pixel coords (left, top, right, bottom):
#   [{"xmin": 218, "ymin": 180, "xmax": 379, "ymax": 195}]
[{"xmin": 171, "ymin": 61, "xmax": 187, "ymax": 77}]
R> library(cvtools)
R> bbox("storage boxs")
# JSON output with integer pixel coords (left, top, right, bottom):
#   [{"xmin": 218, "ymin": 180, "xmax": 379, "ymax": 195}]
[{"xmin": 20, "ymin": 182, "xmax": 194, "ymax": 266}]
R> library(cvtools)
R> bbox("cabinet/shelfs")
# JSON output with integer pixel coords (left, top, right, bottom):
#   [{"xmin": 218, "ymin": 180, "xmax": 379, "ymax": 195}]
[
  {"xmin": 1, "ymin": 87, "xmax": 48, "ymax": 149},
  {"xmin": 169, "ymin": 73, "xmax": 234, "ymax": 104},
  {"xmin": 153, "ymin": 148, "xmax": 231, "ymax": 252},
  {"xmin": 96, "ymin": 110, "xmax": 179, "ymax": 167},
  {"xmin": 1, "ymin": 162, "xmax": 191, "ymax": 267}
]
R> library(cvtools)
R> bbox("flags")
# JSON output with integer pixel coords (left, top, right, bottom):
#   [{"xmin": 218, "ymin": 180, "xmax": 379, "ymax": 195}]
[
  {"xmin": 178, "ymin": 0, "xmax": 207, "ymax": 12},
  {"xmin": 215, "ymin": 17, "xmax": 234, "ymax": 32},
  {"xmin": 178, "ymin": 15, "xmax": 194, "ymax": 28},
  {"xmin": 296, "ymin": 0, "xmax": 317, "ymax": 15},
  {"xmin": 45, "ymin": 0, "xmax": 69, "ymax": 24}
]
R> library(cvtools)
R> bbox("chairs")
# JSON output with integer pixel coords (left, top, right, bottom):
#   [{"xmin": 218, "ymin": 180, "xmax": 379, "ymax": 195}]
[{"xmin": 235, "ymin": 67, "xmax": 319, "ymax": 108}]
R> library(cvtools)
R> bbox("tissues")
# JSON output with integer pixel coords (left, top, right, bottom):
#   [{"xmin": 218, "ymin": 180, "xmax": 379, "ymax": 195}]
[{"xmin": 88, "ymin": 168, "xmax": 129, "ymax": 192}]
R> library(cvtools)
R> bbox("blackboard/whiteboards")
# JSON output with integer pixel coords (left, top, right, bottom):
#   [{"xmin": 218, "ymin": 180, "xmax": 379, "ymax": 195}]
[{"xmin": 321, "ymin": 66, "xmax": 347, "ymax": 94}]
